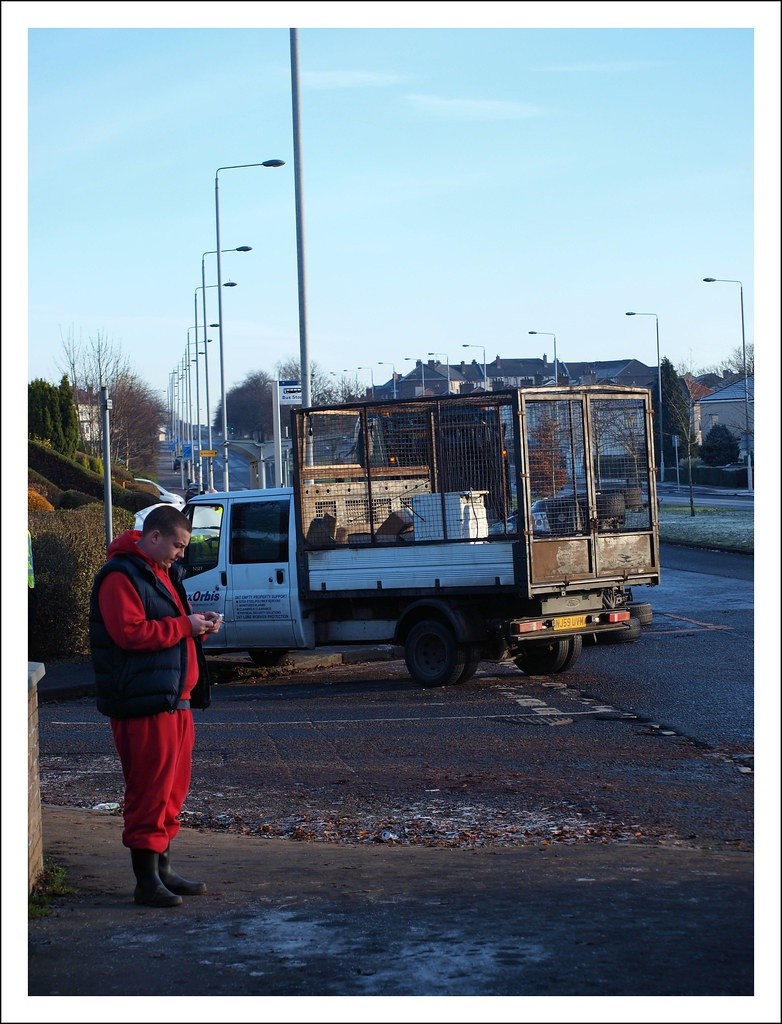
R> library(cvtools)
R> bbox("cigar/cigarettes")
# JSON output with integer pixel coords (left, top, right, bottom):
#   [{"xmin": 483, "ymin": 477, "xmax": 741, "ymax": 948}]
[{"xmin": 221, "ymin": 621, "xmax": 225, "ymax": 624}]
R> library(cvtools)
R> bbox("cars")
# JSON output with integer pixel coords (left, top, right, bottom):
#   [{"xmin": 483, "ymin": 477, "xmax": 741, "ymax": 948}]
[{"xmin": 173, "ymin": 456, "xmax": 189, "ymax": 470}]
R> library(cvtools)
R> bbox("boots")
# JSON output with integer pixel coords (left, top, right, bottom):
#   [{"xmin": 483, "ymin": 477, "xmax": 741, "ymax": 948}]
[
  {"xmin": 130, "ymin": 847, "xmax": 184, "ymax": 905},
  {"xmin": 159, "ymin": 840, "xmax": 206, "ymax": 896}
]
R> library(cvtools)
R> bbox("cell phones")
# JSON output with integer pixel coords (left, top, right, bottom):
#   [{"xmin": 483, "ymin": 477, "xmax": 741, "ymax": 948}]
[{"xmin": 209, "ymin": 613, "xmax": 225, "ymax": 622}]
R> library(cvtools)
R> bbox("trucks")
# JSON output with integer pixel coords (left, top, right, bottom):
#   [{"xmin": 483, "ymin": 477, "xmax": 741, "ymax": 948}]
[{"xmin": 169, "ymin": 382, "xmax": 661, "ymax": 687}]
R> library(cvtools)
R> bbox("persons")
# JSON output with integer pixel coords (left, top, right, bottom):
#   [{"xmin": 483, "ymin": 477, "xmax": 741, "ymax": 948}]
[{"xmin": 89, "ymin": 505, "xmax": 222, "ymax": 907}]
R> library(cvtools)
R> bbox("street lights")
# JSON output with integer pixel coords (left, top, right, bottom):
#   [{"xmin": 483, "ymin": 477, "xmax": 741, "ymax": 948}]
[
  {"xmin": 428, "ymin": 351, "xmax": 451, "ymax": 395},
  {"xmin": 167, "ymin": 323, "xmax": 219, "ymax": 485},
  {"xmin": 343, "ymin": 369, "xmax": 358, "ymax": 391},
  {"xmin": 358, "ymin": 367, "xmax": 374, "ymax": 398},
  {"xmin": 527, "ymin": 331, "xmax": 561, "ymax": 442},
  {"xmin": 378, "ymin": 362, "xmax": 396, "ymax": 399},
  {"xmin": 194, "ymin": 281, "xmax": 238, "ymax": 497},
  {"xmin": 403, "ymin": 357, "xmax": 425, "ymax": 397},
  {"xmin": 213, "ymin": 158, "xmax": 286, "ymax": 492},
  {"xmin": 702, "ymin": 278, "xmax": 752, "ymax": 494},
  {"xmin": 201, "ymin": 245, "xmax": 252, "ymax": 492},
  {"xmin": 625, "ymin": 311, "xmax": 664, "ymax": 483},
  {"xmin": 461, "ymin": 344, "xmax": 486, "ymax": 392}
]
